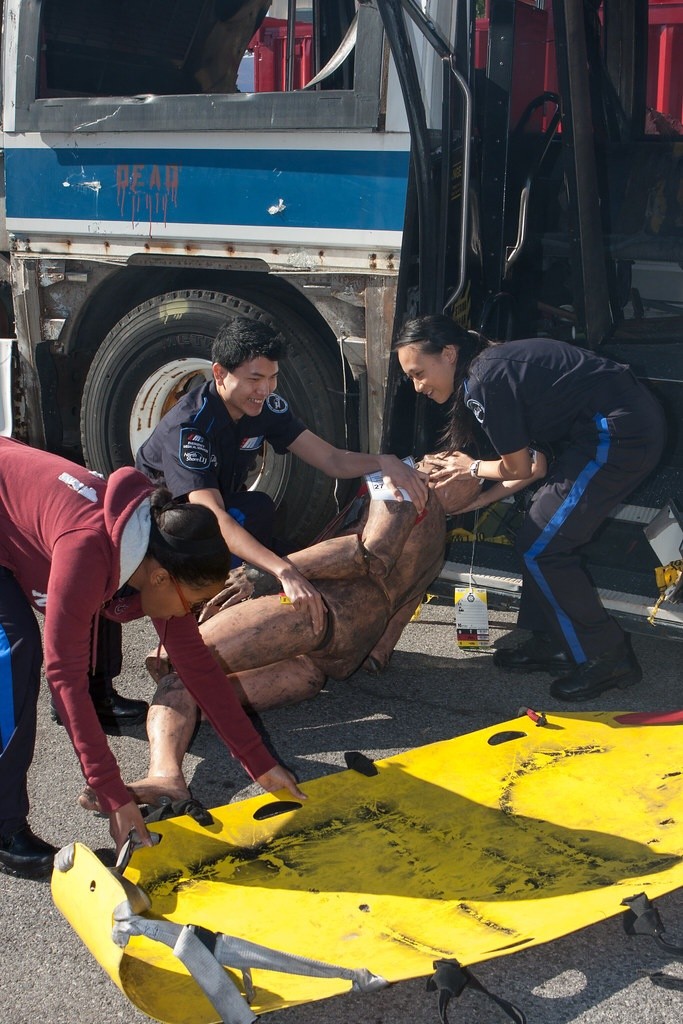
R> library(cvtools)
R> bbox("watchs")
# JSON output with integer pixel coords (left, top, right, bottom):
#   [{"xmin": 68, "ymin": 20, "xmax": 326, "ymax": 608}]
[{"xmin": 470, "ymin": 460, "xmax": 482, "ymax": 480}]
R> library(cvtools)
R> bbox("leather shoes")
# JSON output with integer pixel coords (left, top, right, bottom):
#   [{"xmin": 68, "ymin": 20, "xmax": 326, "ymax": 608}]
[
  {"xmin": 549, "ymin": 633, "xmax": 643, "ymax": 702},
  {"xmin": 492, "ymin": 631, "xmax": 577, "ymax": 675}
]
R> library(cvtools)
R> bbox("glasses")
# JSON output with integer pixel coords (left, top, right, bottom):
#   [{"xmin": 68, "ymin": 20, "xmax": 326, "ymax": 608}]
[{"xmin": 169, "ymin": 571, "xmax": 207, "ymax": 616}]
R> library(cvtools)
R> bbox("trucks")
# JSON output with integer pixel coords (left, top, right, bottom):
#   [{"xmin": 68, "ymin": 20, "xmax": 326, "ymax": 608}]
[{"xmin": 0, "ymin": 1, "xmax": 682, "ymax": 640}]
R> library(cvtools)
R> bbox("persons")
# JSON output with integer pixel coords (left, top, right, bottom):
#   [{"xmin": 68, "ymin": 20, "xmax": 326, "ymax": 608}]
[
  {"xmin": 0, "ymin": 435, "xmax": 308, "ymax": 882},
  {"xmin": 134, "ymin": 317, "xmax": 432, "ymax": 636},
  {"xmin": 78, "ymin": 449, "xmax": 482, "ymax": 811},
  {"xmin": 394, "ymin": 315, "xmax": 670, "ymax": 704}
]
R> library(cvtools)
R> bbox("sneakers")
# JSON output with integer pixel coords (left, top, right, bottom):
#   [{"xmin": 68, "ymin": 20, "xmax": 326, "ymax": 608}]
[
  {"xmin": 51, "ymin": 690, "xmax": 151, "ymax": 728},
  {"xmin": 1, "ymin": 819, "xmax": 61, "ymax": 880}
]
